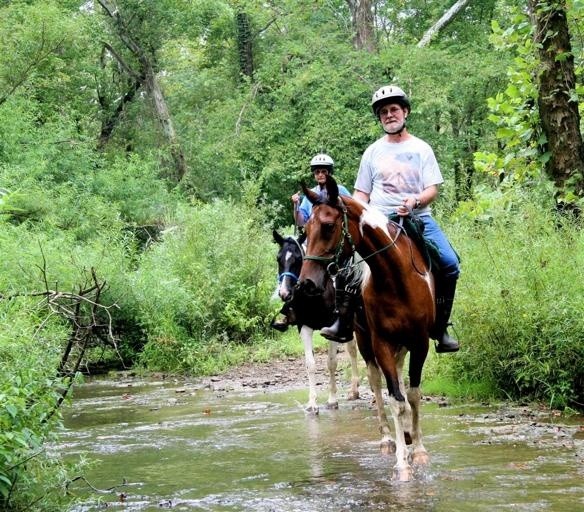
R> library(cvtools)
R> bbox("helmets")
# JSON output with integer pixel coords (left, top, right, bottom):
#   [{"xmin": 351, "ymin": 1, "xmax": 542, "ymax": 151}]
[
  {"xmin": 372, "ymin": 85, "xmax": 410, "ymax": 115},
  {"xmin": 310, "ymin": 153, "xmax": 334, "ymax": 167}
]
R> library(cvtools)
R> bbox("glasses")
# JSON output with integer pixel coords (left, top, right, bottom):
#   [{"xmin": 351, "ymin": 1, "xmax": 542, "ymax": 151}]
[{"xmin": 313, "ymin": 170, "xmax": 328, "ymax": 176}]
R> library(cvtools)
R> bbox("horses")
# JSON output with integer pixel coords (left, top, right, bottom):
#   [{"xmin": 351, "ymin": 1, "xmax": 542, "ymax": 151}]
[
  {"xmin": 296, "ymin": 174, "xmax": 439, "ymax": 483},
  {"xmin": 272, "ymin": 227, "xmax": 377, "ymax": 416}
]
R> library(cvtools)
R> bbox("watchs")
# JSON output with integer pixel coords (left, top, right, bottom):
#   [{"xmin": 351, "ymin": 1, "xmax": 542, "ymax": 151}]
[{"xmin": 415, "ymin": 198, "xmax": 421, "ymax": 210}]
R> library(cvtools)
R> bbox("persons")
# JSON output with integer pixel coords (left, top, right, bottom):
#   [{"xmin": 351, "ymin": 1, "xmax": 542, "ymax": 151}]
[
  {"xmin": 320, "ymin": 84, "xmax": 460, "ymax": 353},
  {"xmin": 274, "ymin": 153, "xmax": 352, "ymax": 325}
]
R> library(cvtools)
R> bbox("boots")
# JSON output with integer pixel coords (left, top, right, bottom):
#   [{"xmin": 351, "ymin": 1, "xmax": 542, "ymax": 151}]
[
  {"xmin": 319, "ymin": 259, "xmax": 357, "ymax": 343},
  {"xmin": 432, "ymin": 276, "xmax": 460, "ymax": 354}
]
